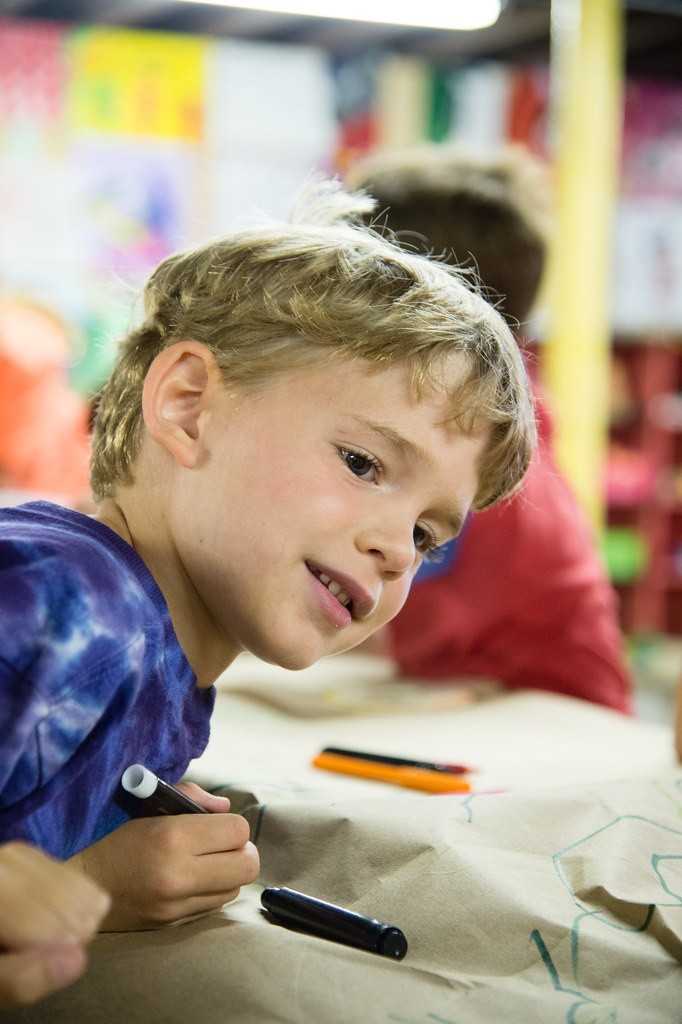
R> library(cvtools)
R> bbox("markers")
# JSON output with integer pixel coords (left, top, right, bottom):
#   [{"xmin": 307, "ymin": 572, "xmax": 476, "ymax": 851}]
[
  {"xmin": 121, "ymin": 763, "xmax": 205, "ymax": 815},
  {"xmin": 260, "ymin": 885, "xmax": 409, "ymax": 961}
]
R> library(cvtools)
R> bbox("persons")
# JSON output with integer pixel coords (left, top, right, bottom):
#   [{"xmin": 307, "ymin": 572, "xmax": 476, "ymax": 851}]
[
  {"xmin": 345, "ymin": 164, "xmax": 634, "ymax": 713},
  {"xmin": 0, "ymin": 224, "xmax": 536, "ymax": 1013}
]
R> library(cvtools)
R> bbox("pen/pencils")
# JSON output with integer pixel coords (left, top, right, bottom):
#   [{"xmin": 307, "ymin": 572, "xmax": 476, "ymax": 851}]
[{"xmin": 313, "ymin": 746, "xmax": 473, "ymax": 794}]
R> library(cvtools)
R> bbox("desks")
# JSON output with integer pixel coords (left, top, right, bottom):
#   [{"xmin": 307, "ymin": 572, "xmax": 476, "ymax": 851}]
[{"xmin": 0, "ymin": 652, "xmax": 682, "ymax": 1024}]
